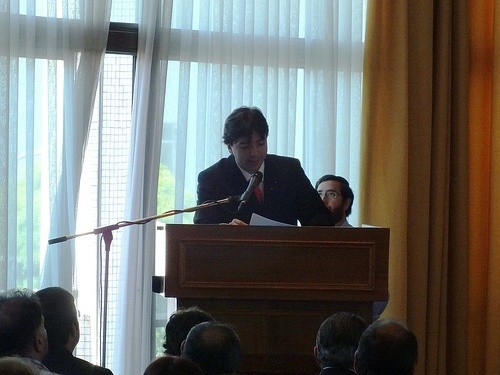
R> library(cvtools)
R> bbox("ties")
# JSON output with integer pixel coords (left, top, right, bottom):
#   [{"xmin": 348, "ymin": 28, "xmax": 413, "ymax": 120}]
[{"xmin": 253, "ymin": 185, "xmax": 263, "ymax": 203}]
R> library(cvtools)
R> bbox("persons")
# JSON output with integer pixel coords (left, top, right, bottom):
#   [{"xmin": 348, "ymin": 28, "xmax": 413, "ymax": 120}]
[
  {"xmin": 315, "ymin": 174, "xmax": 354, "ymax": 227},
  {"xmin": 0, "ymin": 286, "xmax": 419, "ymax": 375},
  {"xmin": 193, "ymin": 106, "xmax": 336, "ymax": 227}
]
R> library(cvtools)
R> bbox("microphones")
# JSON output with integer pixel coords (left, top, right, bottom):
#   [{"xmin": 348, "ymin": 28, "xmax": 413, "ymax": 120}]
[{"xmin": 241, "ymin": 171, "xmax": 263, "ymax": 206}]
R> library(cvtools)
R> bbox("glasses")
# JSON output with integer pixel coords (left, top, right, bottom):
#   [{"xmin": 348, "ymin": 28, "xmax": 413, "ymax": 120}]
[{"xmin": 317, "ymin": 190, "xmax": 343, "ymax": 200}]
